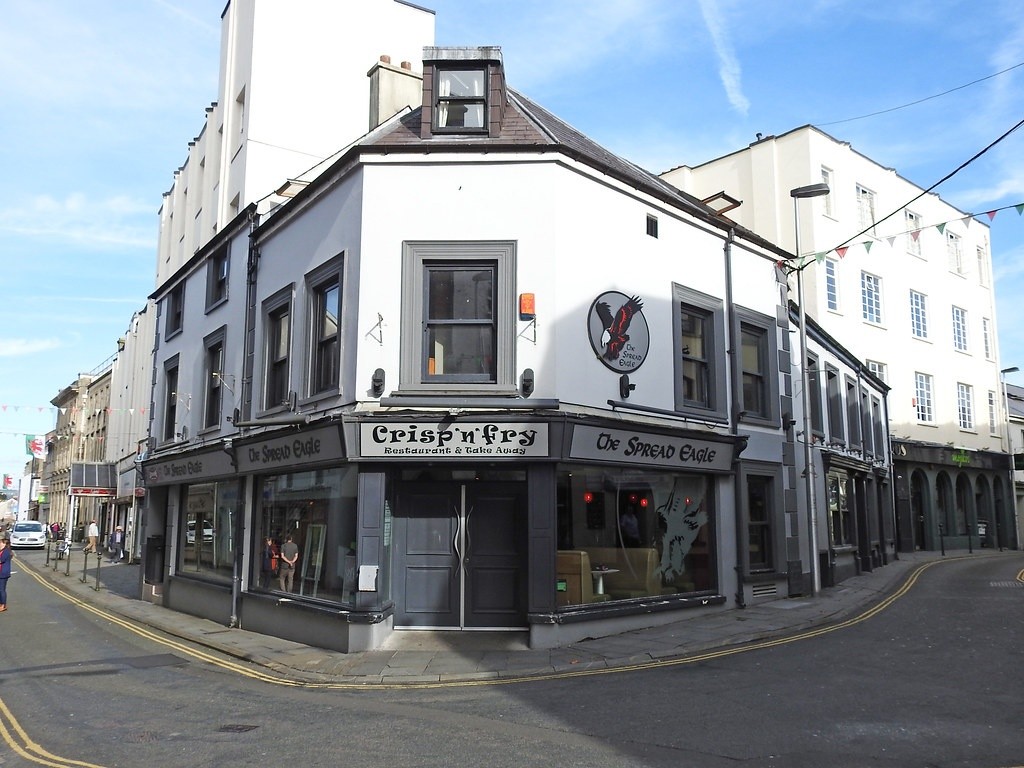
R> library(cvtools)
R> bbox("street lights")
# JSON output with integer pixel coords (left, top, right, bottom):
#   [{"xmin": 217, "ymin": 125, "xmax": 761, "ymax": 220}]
[
  {"xmin": 790, "ymin": 183, "xmax": 831, "ymax": 597},
  {"xmin": 1002, "ymin": 366, "xmax": 1021, "ymax": 551}
]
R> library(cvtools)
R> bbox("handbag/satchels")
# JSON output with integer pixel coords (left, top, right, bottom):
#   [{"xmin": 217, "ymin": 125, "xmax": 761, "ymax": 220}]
[
  {"xmin": 272, "ymin": 567, "xmax": 280, "ymax": 580},
  {"xmin": 108, "ymin": 547, "xmax": 116, "ymax": 553},
  {"xmin": 120, "ymin": 549, "xmax": 123, "ymax": 558}
]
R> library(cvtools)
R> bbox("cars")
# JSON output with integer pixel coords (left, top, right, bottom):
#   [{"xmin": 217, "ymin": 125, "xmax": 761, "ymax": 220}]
[
  {"xmin": 9, "ymin": 521, "xmax": 47, "ymax": 550},
  {"xmin": 186, "ymin": 520, "xmax": 213, "ymax": 545}
]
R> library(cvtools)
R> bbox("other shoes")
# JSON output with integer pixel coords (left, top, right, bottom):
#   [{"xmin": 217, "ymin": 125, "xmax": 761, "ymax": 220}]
[
  {"xmin": 0, "ymin": 609, "xmax": 5, "ymax": 612},
  {"xmin": 0, "ymin": 606, "xmax": 7, "ymax": 610}
]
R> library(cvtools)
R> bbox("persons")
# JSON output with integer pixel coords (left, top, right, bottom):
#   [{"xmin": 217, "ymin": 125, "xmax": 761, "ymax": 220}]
[
  {"xmin": 619, "ymin": 502, "xmax": 641, "ymax": 548},
  {"xmin": 0, "ymin": 518, "xmax": 11, "ymax": 614},
  {"xmin": 263, "ymin": 533, "xmax": 299, "ymax": 595},
  {"xmin": 111, "ymin": 526, "xmax": 125, "ymax": 563},
  {"xmin": 42, "ymin": 520, "xmax": 99, "ymax": 554}
]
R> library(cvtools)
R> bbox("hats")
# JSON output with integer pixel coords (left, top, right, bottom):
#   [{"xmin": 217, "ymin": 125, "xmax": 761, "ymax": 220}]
[
  {"xmin": 116, "ymin": 526, "xmax": 122, "ymax": 529},
  {"xmin": 0, "ymin": 531, "xmax": 10, "ymax": 539},
  {"xmin": 92, "ymin": 520, "xmax": 96, "ymax": 522}
]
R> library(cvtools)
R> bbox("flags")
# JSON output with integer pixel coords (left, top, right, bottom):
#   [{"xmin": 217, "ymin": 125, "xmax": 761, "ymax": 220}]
[
  {"xmin": 26, "ymin": 434, "xmax": 45, "ymax": 459},
  {"xmin": 2, "ymin": 474, "xmax": 13, "ymax": 489}
]
